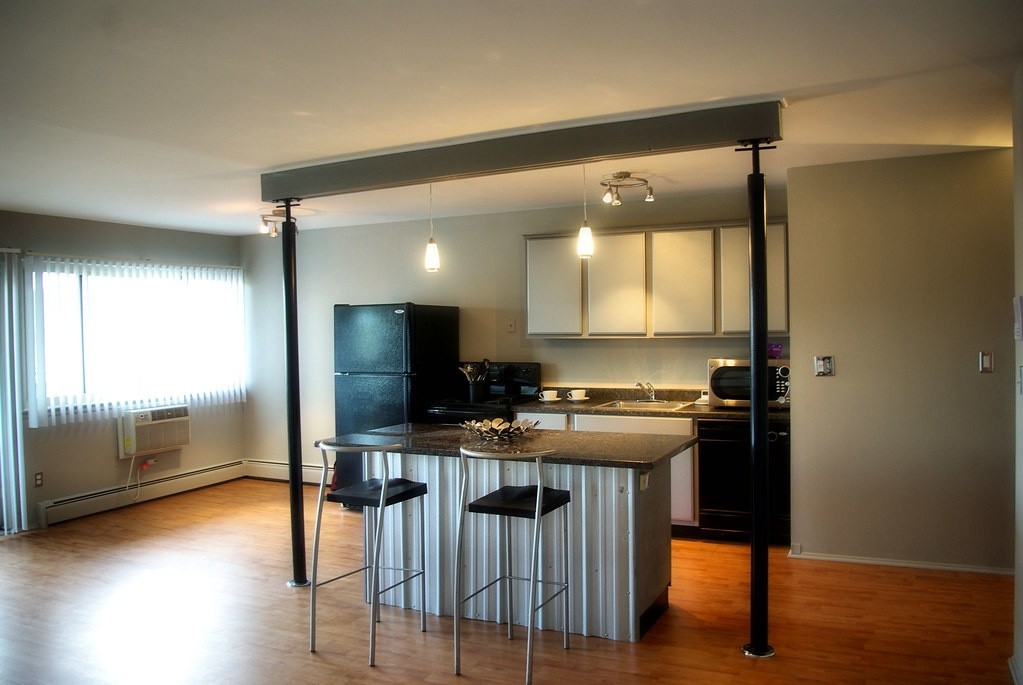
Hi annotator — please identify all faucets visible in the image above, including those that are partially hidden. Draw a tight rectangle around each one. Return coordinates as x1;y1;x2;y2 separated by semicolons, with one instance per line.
634;382;656;400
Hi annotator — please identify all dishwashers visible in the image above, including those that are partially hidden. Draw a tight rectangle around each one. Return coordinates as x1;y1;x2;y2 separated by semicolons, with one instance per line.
697;419;790;540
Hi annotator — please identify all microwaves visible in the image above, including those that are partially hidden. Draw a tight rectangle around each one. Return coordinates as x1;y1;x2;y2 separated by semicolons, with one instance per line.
707;356;790;409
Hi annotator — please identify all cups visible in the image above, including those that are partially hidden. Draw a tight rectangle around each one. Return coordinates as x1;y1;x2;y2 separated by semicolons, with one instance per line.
461;380;482;402
538;390;558;399
567;389;587;399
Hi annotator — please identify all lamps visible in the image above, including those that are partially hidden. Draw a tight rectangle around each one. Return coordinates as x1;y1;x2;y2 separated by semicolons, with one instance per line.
576;161;594;260
600;171;654;205
260;209;297;237
423;182;440;275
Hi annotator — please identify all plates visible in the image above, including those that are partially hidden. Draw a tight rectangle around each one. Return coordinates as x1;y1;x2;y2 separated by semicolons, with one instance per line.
566;397;590;403
538;397;562;404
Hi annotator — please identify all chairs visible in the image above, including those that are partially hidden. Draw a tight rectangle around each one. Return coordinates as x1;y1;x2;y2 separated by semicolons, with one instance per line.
454;447;574;684
310;439;427;667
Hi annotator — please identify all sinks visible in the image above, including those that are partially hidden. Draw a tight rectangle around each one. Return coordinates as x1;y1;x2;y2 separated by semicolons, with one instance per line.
592;400;693;411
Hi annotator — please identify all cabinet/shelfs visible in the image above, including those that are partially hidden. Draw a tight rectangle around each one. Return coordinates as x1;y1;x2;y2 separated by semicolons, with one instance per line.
521;217;791;340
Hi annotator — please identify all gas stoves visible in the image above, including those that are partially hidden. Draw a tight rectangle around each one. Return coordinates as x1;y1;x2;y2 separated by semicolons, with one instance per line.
426;360;541;415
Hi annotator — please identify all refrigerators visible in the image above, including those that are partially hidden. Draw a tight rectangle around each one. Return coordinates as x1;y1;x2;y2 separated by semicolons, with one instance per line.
333;304;460;508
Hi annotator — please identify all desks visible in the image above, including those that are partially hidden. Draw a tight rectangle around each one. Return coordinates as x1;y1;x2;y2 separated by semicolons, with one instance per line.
363;423;699;643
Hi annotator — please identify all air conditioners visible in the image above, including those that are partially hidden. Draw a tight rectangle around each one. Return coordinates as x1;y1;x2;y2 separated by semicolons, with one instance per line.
117;405;192;458
